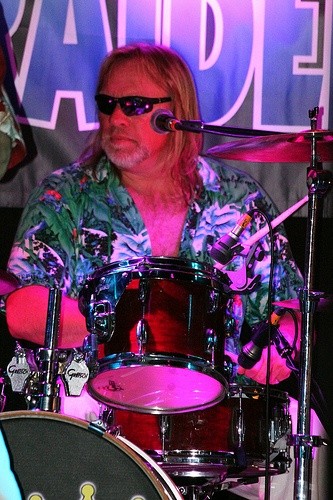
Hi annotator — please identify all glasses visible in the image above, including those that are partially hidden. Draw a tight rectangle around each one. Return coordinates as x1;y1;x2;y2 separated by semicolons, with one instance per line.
95;93;173;117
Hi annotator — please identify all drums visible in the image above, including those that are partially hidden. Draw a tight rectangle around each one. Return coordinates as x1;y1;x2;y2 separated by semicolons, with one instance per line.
222;382;291;479
76;256;232;414
1;410;182;500
101;407;232;479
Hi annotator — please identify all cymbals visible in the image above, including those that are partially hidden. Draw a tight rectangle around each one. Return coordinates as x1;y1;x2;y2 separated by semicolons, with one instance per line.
206;129;333;163
271;296;333;315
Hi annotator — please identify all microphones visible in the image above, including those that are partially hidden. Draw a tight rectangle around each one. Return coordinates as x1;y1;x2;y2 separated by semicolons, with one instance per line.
209;210;255;265
150;108;182;134
238;308;286;370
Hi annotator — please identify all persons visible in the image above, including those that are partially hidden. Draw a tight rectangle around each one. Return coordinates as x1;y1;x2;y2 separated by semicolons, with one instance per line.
0;42;327;500
